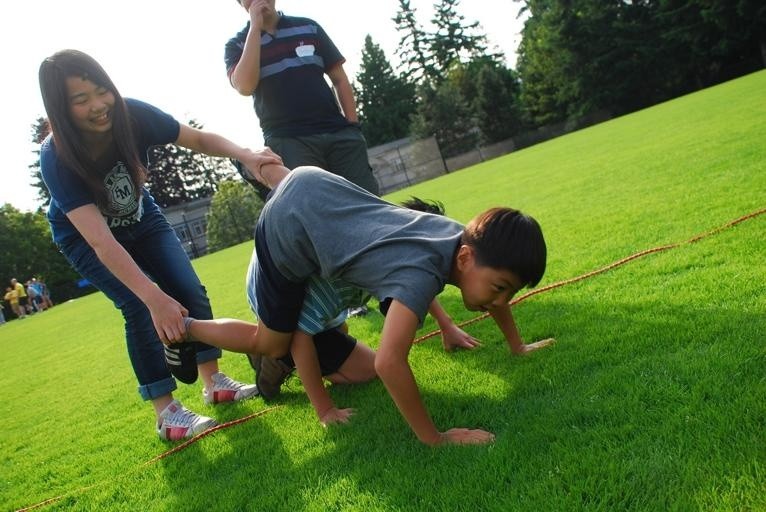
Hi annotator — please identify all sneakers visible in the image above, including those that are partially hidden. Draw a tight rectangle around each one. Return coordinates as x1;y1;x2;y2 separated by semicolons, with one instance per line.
348;305;370;317
248;354;295;404
163;337;198;385
229;156;272;203
155;399;219;442
202;371;259;405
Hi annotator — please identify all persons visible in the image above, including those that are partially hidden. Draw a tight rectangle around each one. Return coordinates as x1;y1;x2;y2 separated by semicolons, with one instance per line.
0;274;53;324
244;195;485;403
38;49;285;441
161;151;558;446
222;0;384;317
